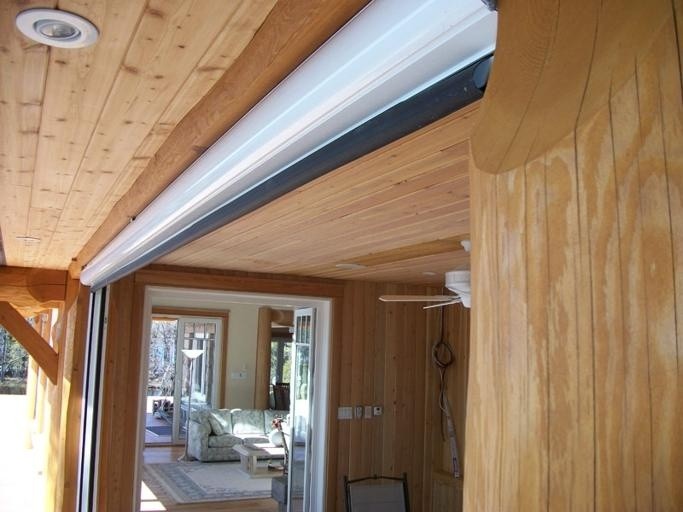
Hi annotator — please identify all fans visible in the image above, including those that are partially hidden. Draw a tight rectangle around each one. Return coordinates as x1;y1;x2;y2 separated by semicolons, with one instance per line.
379;241;471;310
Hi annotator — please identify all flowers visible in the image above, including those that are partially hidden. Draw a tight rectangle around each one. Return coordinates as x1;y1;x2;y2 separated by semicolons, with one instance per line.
271;418;284;430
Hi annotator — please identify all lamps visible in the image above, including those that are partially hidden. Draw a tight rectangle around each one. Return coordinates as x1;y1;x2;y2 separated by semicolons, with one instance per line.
177;349;206;460
18;9;99;48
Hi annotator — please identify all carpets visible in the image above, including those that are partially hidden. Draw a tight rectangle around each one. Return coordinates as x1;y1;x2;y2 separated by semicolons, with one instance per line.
145;426;187;435
142;462;288;504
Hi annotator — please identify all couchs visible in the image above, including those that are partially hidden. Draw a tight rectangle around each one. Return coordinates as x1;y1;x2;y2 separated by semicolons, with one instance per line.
187;408;290;461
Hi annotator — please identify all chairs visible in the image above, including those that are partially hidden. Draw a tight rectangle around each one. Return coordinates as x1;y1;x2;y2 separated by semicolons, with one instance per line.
344;472;411;512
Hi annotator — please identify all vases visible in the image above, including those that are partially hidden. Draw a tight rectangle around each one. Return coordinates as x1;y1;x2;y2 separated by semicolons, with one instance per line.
268;429;284;447
